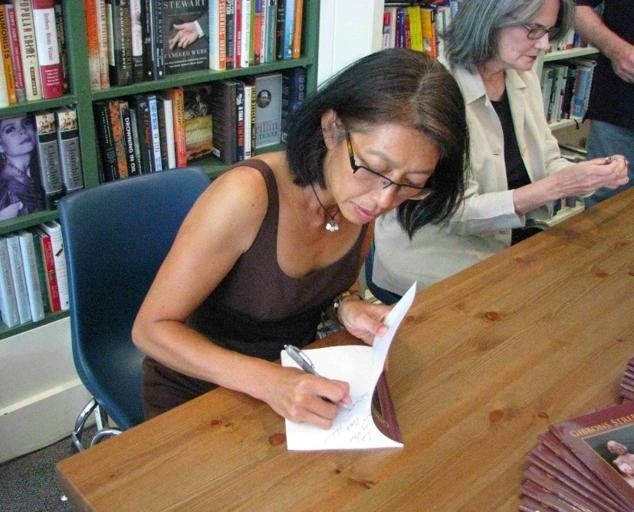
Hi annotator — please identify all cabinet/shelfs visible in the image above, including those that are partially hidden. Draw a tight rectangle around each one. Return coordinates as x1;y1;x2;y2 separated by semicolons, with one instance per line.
0;1;321;464
372;0;605;133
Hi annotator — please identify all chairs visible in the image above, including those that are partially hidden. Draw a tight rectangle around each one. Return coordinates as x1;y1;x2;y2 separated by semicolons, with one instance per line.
56;165;212;459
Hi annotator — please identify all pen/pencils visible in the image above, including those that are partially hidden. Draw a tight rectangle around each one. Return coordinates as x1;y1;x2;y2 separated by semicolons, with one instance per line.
604;156;611;164
283;344;352;413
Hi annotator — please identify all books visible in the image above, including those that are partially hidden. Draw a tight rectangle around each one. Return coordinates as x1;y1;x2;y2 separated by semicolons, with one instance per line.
0;220;71;328
514;353;633;511
0;1;303;106
0;68;309;221
377;1;597;141
272;280;424;451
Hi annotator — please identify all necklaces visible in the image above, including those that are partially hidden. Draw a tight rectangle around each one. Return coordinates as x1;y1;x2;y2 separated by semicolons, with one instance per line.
306;178;343;234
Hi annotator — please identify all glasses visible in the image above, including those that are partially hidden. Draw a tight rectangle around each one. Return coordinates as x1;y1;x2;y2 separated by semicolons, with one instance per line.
508;14;560;41
340;113;435;200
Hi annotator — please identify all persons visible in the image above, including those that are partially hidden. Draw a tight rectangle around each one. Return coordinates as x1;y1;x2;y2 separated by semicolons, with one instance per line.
130;44;469;434
569;0;633;210
361;1;631;306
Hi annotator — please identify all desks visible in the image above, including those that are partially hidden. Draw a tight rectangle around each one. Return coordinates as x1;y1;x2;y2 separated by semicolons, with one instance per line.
52;185;634;510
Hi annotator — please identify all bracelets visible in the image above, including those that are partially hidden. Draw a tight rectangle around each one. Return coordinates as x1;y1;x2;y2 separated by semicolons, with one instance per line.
331;289;361;327
